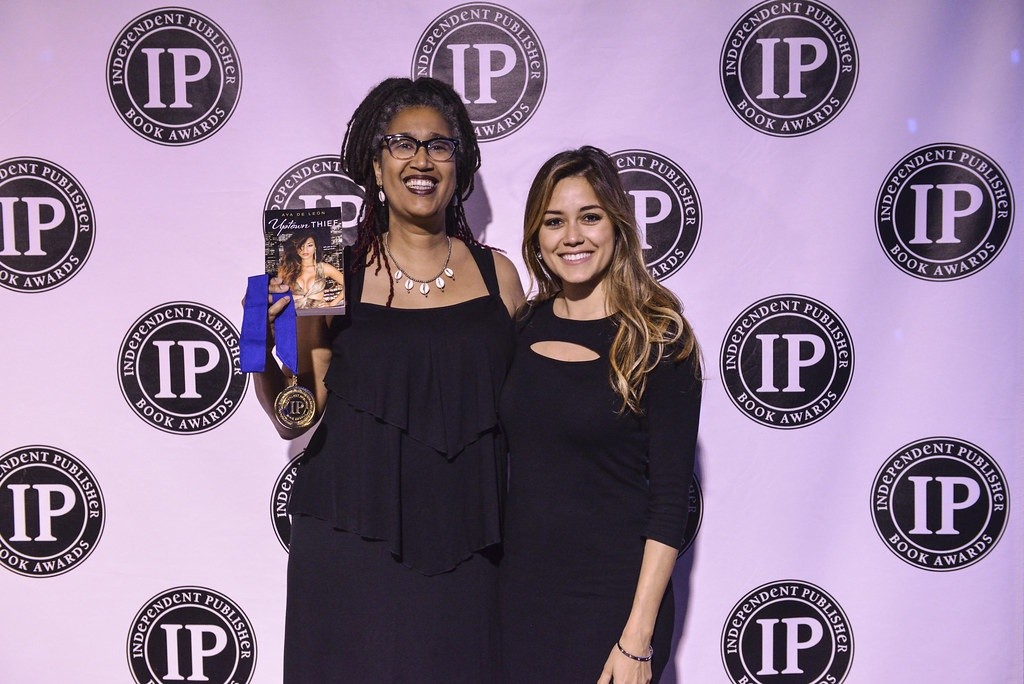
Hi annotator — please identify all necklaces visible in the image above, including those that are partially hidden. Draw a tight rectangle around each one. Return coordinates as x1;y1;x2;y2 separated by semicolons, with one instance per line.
379;234;458;298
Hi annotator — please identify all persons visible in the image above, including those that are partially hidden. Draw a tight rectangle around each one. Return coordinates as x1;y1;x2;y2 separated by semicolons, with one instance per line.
514;145;703;683
251;77;528;683
277;232;345;308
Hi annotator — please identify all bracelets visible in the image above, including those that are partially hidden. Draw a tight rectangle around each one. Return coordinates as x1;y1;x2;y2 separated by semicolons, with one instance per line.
615;639;655;664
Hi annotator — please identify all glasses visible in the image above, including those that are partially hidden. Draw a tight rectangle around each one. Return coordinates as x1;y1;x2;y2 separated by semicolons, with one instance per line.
377;134;461;163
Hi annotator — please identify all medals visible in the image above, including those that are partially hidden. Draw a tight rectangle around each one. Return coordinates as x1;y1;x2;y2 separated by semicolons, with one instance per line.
274;384;316;429
